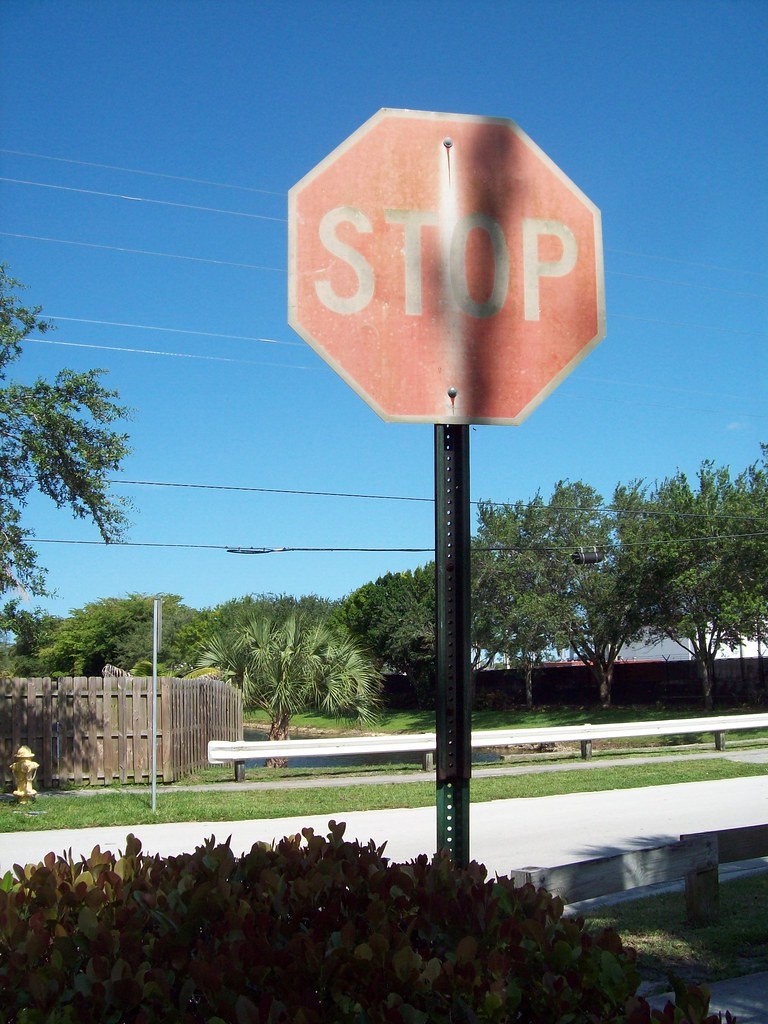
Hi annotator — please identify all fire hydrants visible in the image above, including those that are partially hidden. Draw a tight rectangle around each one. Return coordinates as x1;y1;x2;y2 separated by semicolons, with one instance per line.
9;745;41;807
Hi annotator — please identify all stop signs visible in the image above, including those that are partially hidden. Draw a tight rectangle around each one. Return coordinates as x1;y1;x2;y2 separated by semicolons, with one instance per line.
282;107;607;424
